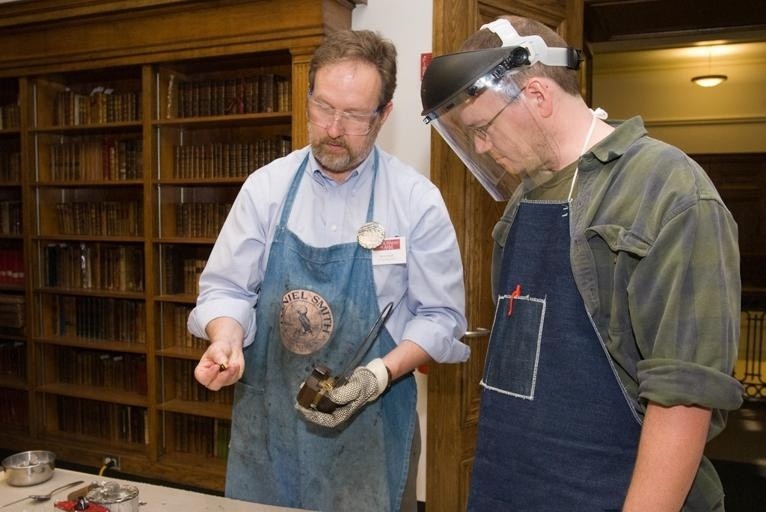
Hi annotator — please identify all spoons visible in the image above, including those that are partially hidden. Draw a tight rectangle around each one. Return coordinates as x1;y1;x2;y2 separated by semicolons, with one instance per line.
30;480;84;501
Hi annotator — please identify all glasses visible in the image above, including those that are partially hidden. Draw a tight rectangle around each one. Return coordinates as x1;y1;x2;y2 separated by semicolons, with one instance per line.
464;87;533;145
306;89;383;135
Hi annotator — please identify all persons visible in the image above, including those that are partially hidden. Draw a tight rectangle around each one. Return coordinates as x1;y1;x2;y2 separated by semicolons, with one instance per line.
417;14;744;511
185;29;473;512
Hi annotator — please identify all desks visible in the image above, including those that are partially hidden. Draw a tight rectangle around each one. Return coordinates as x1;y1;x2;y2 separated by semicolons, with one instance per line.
0;468;317;512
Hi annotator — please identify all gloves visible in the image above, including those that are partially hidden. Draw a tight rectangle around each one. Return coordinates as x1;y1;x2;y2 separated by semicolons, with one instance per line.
294;359;388;428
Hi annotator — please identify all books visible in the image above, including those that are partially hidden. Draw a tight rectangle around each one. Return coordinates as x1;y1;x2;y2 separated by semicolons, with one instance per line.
172;259;235;462
49;91;143;448
172;74;292;118
172;139;292;177
0;104;29;437
176;186;232;238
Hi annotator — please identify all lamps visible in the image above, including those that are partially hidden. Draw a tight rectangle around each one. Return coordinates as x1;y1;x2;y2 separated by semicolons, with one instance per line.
691;75;728;88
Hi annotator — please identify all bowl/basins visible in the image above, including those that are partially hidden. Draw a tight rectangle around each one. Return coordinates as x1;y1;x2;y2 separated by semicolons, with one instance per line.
2;450;56;487
